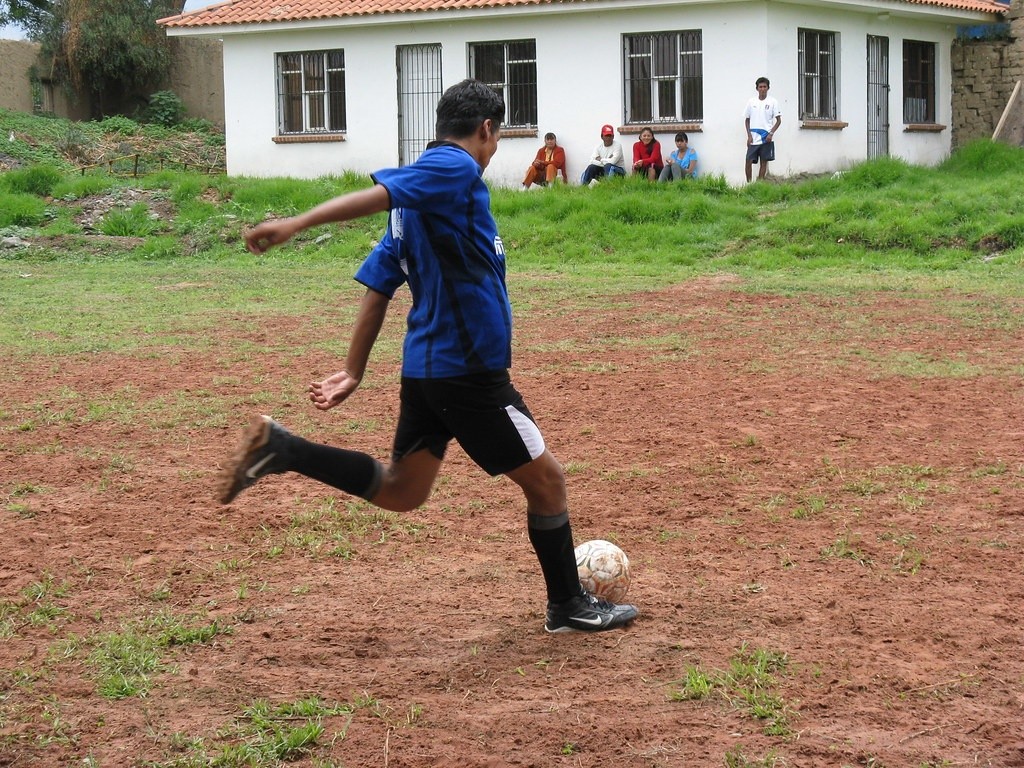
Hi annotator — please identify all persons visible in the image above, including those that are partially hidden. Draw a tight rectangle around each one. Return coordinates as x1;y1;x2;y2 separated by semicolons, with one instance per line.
744;76;781;184
578;124;627;187
219;78;639;633
521;132;568;193
632;127;664;180
658;131;699;182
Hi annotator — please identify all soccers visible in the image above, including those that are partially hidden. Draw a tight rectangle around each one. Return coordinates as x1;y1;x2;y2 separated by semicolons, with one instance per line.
574;539;631;605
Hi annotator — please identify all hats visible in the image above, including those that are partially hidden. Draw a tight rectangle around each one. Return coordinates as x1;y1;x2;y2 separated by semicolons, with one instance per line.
603;125;613;136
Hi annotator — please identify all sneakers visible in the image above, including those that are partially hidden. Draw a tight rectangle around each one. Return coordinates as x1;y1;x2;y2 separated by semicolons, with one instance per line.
214;415;290;505
544;583;639;633
